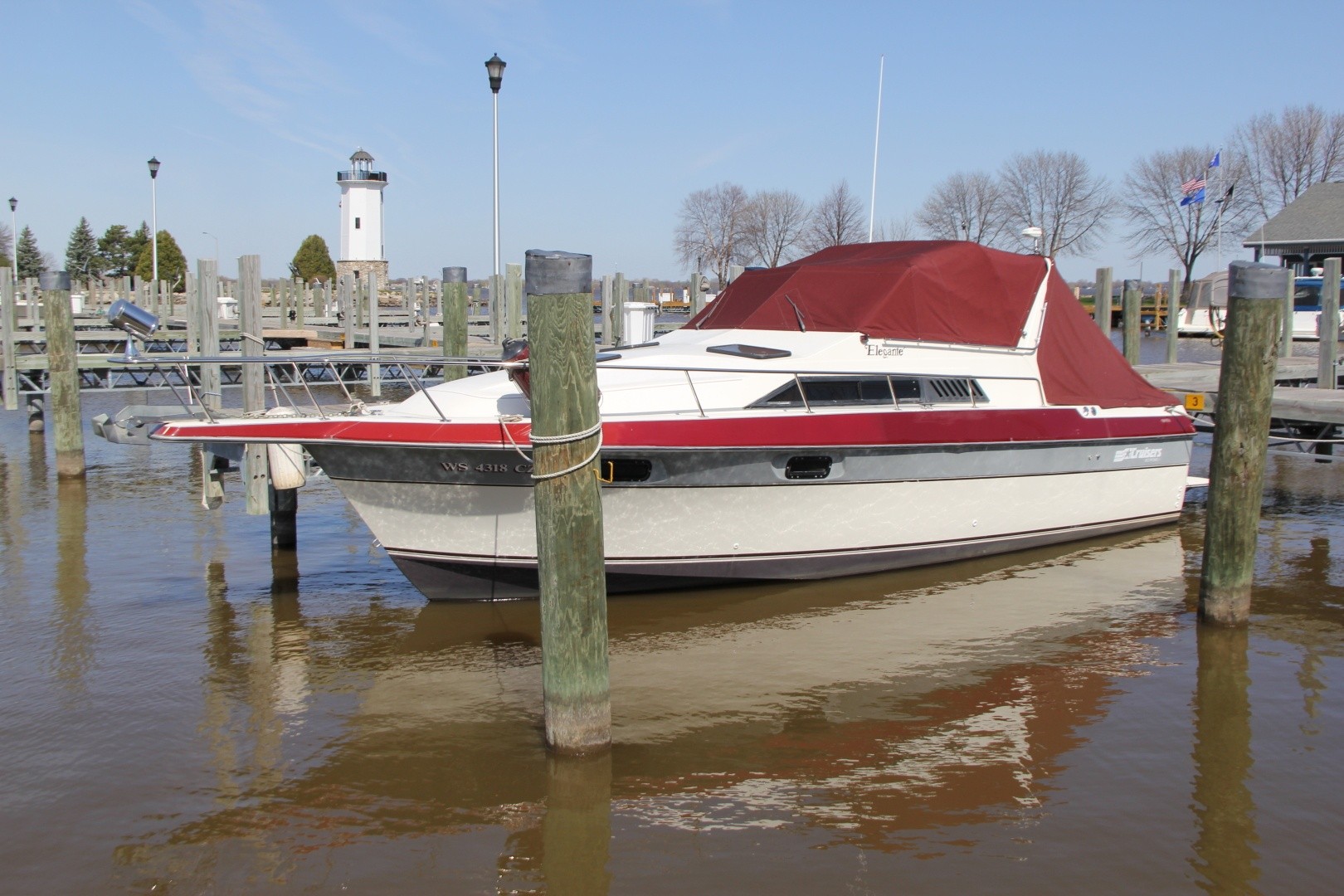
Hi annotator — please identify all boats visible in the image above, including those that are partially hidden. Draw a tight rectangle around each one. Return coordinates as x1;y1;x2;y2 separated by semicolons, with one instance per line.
144;238;1214;604
1279;267;1344;343
1176;269;1229;337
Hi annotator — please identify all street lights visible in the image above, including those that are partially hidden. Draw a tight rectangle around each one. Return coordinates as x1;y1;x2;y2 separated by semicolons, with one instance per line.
147;156;161;329
483;52;506;344
8;196;20;303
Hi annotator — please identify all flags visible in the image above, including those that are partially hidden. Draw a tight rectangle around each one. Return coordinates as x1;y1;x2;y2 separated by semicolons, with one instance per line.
1208;152;1221;168
1180;186;1206;207
1181;170;1206;194
1215;183;1235;203
